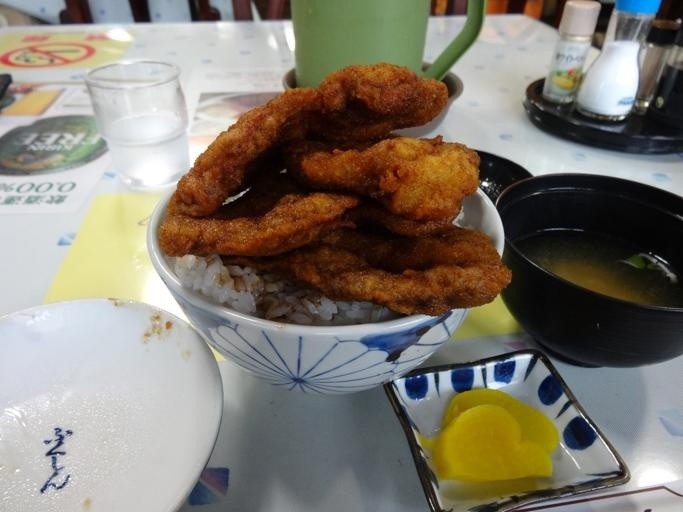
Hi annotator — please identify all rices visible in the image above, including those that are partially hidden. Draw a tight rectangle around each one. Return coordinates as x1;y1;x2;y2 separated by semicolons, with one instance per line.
172;204;466;325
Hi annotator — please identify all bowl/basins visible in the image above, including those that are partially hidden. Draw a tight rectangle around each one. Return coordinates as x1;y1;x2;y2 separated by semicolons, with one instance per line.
493;173;683;368
280;64;463;140
146;188;505;394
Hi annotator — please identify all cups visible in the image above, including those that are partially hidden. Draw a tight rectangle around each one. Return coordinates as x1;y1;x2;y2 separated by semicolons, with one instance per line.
85;60;190;191
289;1;484;89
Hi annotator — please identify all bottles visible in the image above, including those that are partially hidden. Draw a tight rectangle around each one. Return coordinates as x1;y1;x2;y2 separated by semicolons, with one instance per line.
631;20;678;116
654;35;683;112
574;0;661;121
542;0;601;105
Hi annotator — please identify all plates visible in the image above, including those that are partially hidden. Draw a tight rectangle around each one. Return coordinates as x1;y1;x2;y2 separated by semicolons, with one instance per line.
0;299;223;511
384;348;630;511
